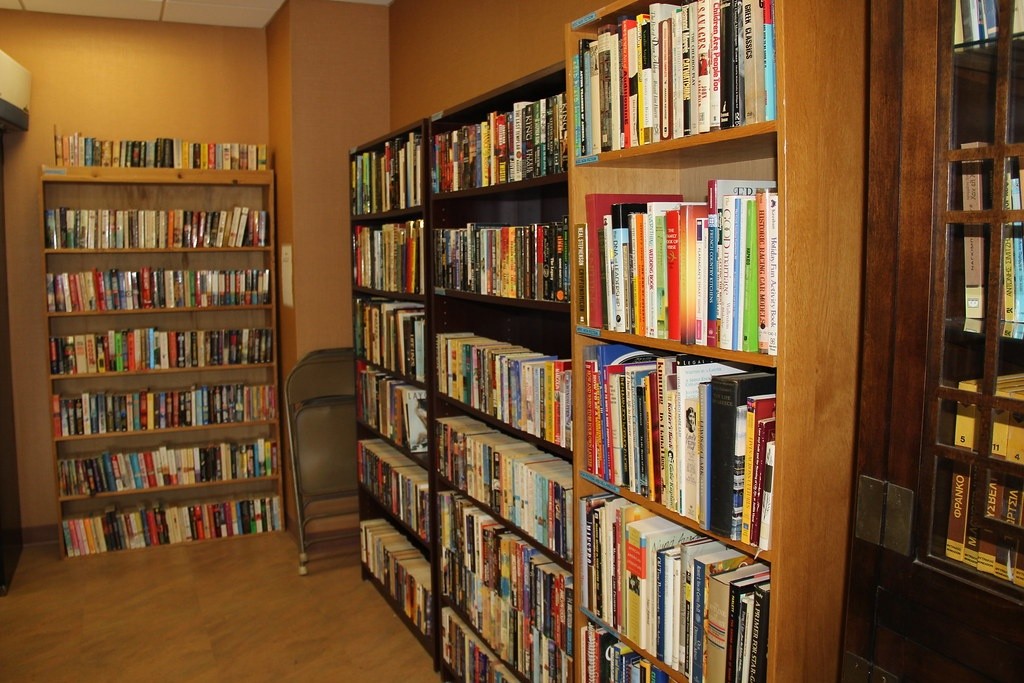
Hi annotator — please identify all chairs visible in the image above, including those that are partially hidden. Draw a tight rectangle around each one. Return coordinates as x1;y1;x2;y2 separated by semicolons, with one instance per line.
285;347;361;575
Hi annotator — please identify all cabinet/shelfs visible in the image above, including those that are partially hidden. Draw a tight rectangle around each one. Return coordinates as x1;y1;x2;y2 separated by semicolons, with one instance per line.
347;115;440;675
38;162;288;559
564;0;840;683
851;0;1023;682
427;60;574;683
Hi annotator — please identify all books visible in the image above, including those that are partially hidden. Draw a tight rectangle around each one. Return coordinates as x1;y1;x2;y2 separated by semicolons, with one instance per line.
571;0;777;158
349;132;432;637
46;269;270;312
49;327;272;374
574;180;777;356
52;383;275;437
435;416;574;683
55;132;267;170
945;374;1024;587
579;492;770;683
46;207;266;248
580;622;677;683
57;439;278;497
961;0;998;50
435;332;573;452
63;491;282;557
961;141;1024;320
583;345;776;551
433;215;570;303
431;91;568;193
